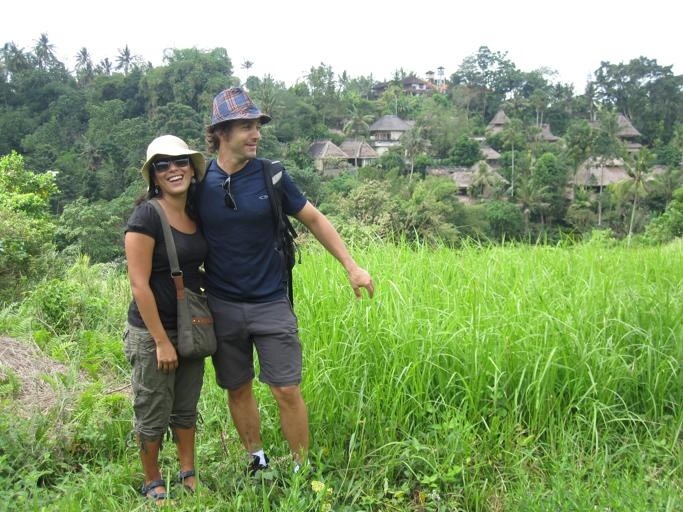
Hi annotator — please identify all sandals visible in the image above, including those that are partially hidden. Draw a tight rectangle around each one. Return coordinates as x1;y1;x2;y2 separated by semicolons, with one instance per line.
141;470;214;508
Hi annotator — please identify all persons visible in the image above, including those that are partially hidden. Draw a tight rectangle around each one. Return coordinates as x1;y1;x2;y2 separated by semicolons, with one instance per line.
191;86;377;490
121;131;218;503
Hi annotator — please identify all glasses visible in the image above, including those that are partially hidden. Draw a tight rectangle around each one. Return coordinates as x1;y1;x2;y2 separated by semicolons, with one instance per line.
222;176;238;211
151;156;191;173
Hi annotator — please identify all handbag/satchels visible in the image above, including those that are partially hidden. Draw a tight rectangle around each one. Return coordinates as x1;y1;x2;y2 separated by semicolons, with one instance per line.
176;288;217;358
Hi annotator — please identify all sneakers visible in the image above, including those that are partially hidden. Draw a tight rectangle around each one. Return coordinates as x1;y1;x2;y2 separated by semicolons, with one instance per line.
232;453;273;494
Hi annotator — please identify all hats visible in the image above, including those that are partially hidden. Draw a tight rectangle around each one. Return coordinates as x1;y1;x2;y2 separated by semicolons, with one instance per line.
141;134;206;183
210;86;272;128
